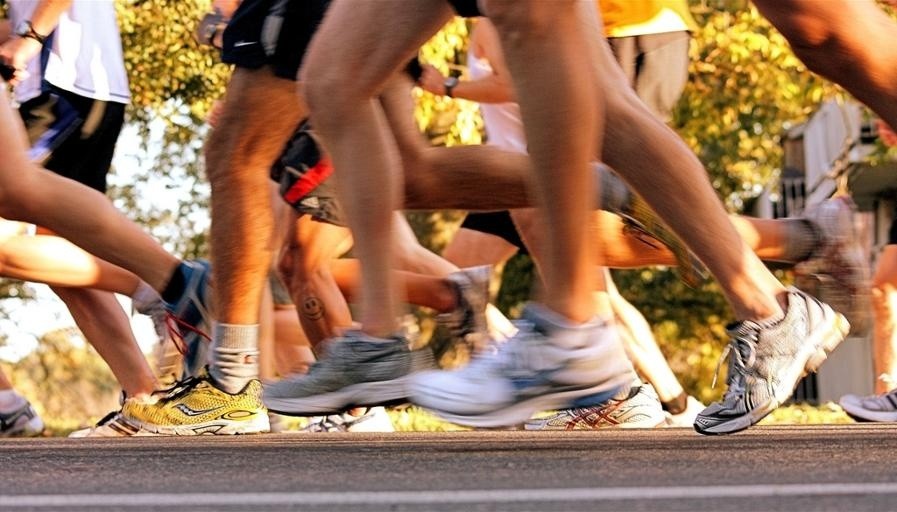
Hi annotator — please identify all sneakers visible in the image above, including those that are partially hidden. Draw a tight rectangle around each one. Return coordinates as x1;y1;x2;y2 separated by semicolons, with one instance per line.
130;294;183;387
122;364;270;434
164;259;215;376
3;401;44;433
693;284;851;432
261;208;683;434
70;387;160;439
786;199;871;341
619;173;708;285
840;392;896;425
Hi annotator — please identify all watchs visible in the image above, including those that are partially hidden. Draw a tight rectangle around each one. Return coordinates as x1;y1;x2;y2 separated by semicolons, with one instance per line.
16;19;47;45
444;77;458;98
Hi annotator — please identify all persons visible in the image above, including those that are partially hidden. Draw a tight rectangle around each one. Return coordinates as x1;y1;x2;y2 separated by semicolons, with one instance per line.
2;0;212;441
758;1;897;434
128;1;851;435
